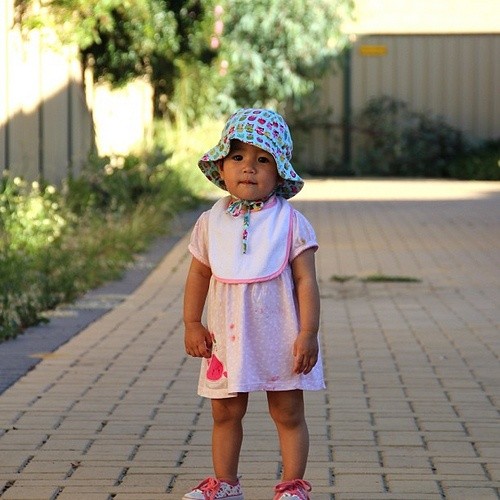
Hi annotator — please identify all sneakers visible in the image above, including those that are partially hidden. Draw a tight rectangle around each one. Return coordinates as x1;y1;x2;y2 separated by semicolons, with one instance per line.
273;479;312;500
182;477;243;500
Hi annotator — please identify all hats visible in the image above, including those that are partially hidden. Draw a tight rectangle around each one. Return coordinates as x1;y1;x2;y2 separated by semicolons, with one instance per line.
198;108;304;200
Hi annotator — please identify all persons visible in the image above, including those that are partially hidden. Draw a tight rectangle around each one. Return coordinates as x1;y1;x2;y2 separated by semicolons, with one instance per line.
182;108;326;500
